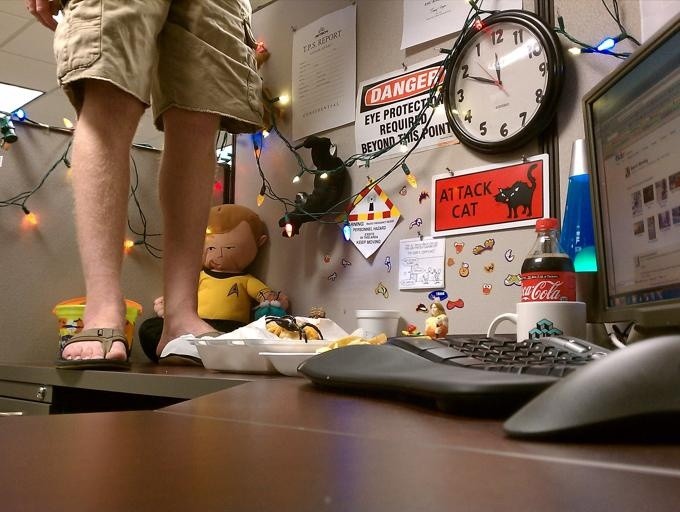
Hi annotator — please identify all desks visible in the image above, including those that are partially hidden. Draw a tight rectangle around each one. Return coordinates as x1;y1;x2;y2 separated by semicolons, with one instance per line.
0;359;680;512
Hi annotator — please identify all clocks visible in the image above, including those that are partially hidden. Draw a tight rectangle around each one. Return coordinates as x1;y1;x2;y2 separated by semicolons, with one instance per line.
443;8;565;155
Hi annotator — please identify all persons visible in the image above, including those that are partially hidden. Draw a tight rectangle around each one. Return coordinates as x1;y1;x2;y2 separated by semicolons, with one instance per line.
424;299;451;339
137;205;289;360
22;0;271;372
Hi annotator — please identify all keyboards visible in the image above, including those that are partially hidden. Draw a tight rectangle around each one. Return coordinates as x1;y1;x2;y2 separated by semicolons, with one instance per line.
295;335;614;414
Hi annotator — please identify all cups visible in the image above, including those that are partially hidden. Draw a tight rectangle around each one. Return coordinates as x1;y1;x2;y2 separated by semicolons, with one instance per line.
484;299;589;343
355;308;402;340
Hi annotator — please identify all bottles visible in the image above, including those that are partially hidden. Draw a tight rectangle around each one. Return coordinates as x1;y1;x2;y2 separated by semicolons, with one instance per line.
518;216;580;302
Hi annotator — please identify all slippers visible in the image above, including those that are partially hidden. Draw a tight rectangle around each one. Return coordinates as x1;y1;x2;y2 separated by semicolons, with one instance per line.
54;328;132;370
158;332;227;367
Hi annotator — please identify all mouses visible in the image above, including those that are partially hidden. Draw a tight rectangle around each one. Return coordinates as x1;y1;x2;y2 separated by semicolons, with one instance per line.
503;333;680;439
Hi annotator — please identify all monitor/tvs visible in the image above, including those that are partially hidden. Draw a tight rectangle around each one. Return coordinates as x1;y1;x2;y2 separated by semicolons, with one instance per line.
580;12;680;331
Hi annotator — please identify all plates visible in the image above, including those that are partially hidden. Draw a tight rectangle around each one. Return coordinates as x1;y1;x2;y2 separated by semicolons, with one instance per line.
255;349;320;376
